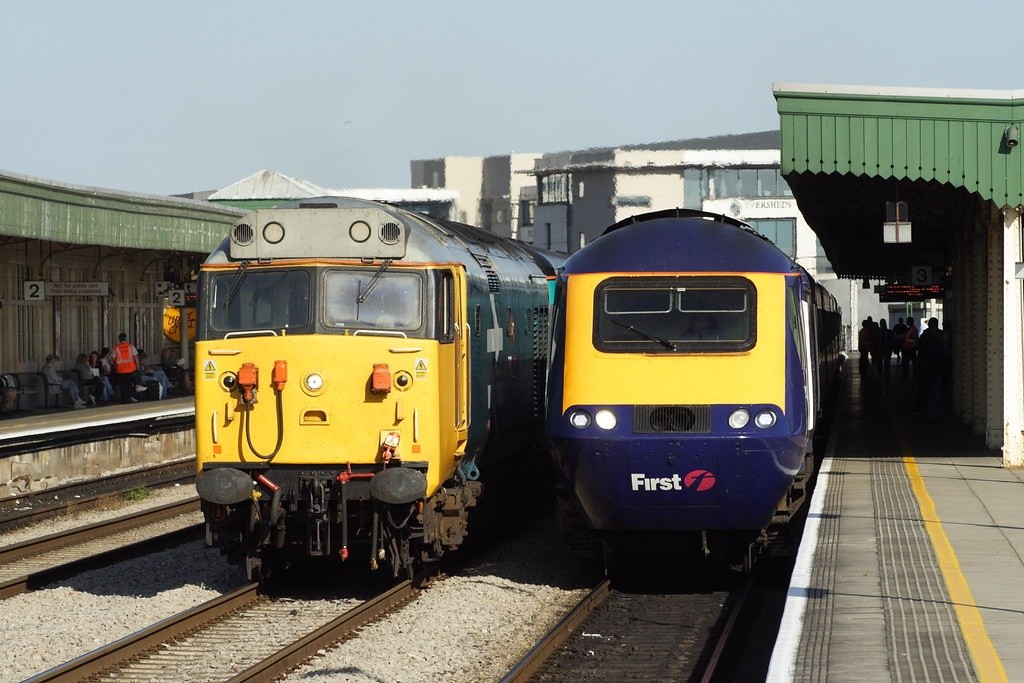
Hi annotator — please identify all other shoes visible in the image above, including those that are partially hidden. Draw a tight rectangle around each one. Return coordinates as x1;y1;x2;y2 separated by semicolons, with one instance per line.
77;397;87;405
128;396;138;403
74;402;87;410
136;384;148;393
88;394;97;408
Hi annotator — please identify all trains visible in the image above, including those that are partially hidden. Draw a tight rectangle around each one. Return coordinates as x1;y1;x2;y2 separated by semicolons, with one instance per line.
543;207;843;584
194;197;576;587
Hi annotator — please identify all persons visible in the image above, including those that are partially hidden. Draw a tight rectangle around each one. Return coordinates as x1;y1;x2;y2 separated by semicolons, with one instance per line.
858;314;952;415
75;334;189;407
40;354;86;409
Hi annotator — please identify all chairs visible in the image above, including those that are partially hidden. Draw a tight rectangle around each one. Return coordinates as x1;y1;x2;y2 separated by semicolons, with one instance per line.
37;371;71;410
69;368;98;404
0;373;38;413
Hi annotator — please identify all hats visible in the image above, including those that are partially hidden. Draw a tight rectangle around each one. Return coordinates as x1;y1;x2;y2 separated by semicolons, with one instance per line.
45;353;54;364
